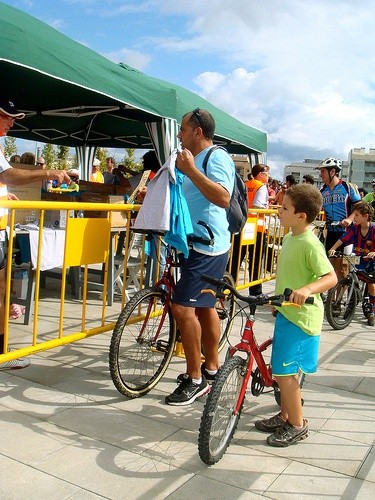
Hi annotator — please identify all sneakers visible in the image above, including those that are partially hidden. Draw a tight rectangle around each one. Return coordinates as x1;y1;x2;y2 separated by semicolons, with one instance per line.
176;362;221;386
254;412;289;433
165;373;211;407
266;419;310;448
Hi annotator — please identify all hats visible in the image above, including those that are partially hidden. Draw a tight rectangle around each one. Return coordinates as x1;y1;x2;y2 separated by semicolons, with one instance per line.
0;98;26;120
92;158;101;167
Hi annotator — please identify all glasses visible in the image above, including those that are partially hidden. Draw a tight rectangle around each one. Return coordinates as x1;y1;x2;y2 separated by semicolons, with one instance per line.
142;161;145;164
0;114;16;122
261;172;270;176
302;180;306;183
106;161;110;164
192;106;205;130
36;162;45;167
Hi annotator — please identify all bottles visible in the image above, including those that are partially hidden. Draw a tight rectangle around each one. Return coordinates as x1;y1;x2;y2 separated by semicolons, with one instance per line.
77;210;84;218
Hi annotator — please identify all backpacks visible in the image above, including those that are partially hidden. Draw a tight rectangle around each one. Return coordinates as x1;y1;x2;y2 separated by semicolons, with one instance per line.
201;145;249;235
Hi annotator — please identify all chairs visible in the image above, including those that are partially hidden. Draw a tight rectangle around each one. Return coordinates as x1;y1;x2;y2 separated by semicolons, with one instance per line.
98;232;151;301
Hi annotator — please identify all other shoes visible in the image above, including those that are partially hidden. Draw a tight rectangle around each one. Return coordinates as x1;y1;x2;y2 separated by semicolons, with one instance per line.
348;295;357;308
366;303;375;326
0;354;31;371
331;302;341;317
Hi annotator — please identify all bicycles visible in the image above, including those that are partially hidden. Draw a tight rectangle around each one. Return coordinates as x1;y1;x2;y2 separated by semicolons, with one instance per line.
108;218;238;400
325;250;375;331
196;271;314;465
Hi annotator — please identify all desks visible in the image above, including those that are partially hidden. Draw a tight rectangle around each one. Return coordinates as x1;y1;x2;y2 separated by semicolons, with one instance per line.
9;226;131;326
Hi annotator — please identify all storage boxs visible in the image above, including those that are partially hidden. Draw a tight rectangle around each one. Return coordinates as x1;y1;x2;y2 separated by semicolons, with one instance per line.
45;209;74;228
42;192;81;202
7;159;43;227
81;193;128;227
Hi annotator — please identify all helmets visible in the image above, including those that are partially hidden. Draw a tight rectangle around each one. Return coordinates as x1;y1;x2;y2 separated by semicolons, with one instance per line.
314;157;342;170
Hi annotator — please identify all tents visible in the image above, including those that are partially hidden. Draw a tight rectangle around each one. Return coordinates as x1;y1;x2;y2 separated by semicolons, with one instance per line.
0;1;268;287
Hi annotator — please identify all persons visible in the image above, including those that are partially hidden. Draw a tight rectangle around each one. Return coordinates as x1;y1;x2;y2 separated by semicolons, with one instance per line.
164;107;236;406
255;182;339;448
0;99;375;328
